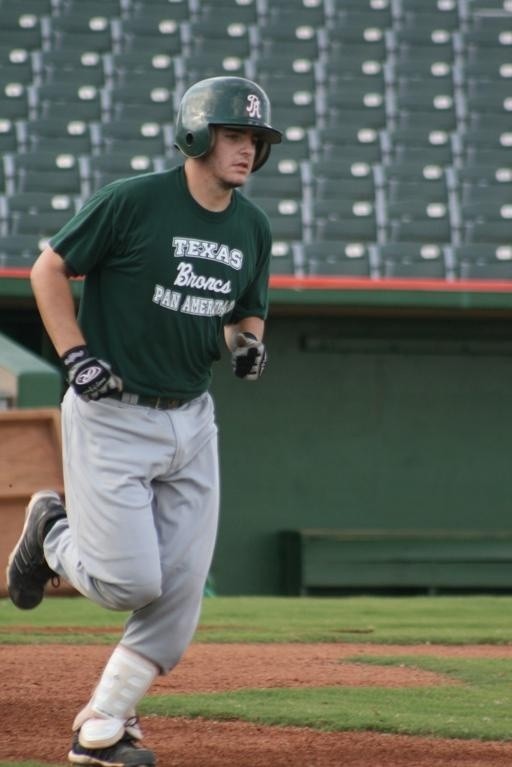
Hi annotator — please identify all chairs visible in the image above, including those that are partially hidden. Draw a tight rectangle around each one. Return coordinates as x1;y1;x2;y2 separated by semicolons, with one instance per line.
0;0;511;279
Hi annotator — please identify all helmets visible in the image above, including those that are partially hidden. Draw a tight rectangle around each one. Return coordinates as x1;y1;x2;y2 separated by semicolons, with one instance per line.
173;75;283;173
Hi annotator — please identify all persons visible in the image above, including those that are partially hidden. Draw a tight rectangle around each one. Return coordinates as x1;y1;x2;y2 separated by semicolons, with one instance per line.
0;73;287;767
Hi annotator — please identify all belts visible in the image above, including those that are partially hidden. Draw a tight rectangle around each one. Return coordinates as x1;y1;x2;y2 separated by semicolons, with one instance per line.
105;390;189;413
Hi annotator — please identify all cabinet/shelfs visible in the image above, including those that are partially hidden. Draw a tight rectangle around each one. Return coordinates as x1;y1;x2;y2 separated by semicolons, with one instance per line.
0;406;65;597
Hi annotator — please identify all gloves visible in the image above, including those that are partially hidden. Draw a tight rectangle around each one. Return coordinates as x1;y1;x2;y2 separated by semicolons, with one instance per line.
230;330;269;382
61;344;124;402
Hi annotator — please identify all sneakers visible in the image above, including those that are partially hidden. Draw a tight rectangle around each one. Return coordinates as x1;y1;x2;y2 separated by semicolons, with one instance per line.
67;707;157;767
6;489;68;610
